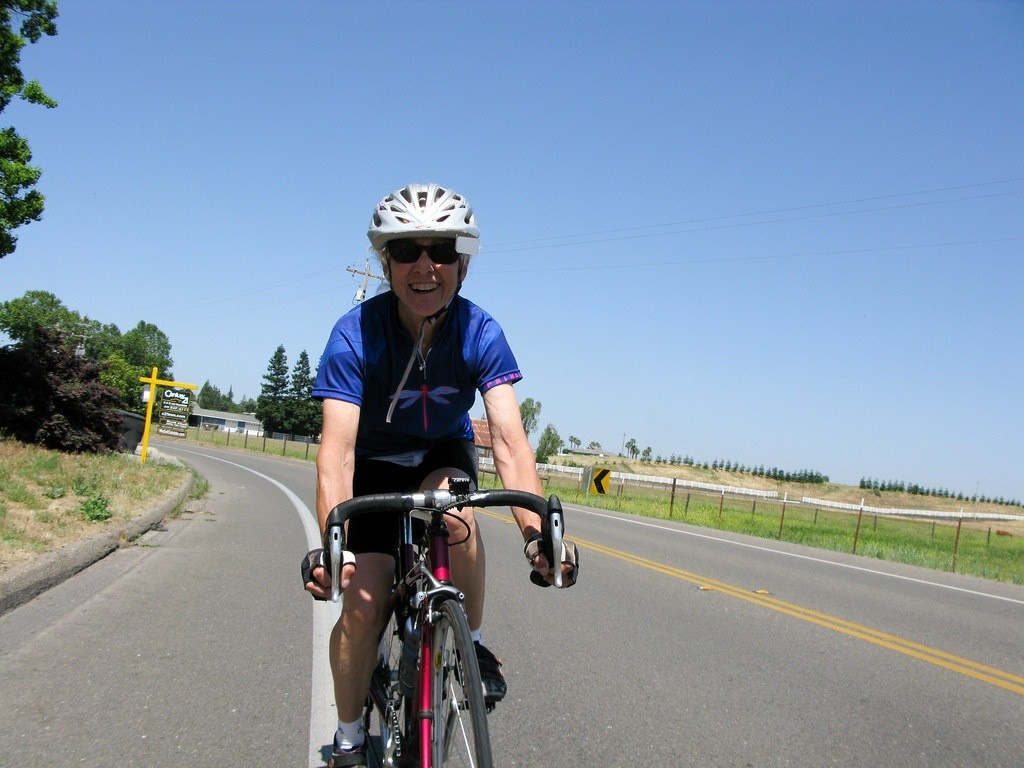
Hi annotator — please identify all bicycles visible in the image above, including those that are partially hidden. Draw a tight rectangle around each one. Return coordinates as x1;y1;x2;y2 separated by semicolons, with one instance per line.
320;486;577;768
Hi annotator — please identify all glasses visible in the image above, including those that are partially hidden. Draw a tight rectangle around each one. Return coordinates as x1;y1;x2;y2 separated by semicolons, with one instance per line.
382;239;463;264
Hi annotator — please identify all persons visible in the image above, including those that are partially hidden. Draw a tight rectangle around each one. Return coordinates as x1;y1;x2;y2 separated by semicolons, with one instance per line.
301;184;580;768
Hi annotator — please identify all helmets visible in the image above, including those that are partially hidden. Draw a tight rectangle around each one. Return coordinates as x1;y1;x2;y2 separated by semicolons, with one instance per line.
368;184;480;265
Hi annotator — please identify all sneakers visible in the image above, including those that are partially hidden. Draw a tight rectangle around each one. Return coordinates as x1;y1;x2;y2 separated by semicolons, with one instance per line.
328;732;372;768
452;643;508;702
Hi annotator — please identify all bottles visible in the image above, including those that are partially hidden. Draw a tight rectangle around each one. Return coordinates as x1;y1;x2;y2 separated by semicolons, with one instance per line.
399;601;425;696
